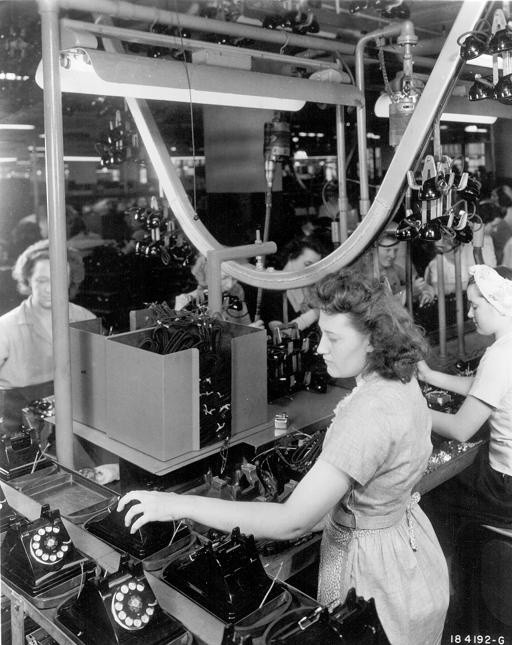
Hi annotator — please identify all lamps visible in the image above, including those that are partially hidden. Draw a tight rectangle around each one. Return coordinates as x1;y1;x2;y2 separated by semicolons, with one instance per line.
375;71;512;125
34;16;366;113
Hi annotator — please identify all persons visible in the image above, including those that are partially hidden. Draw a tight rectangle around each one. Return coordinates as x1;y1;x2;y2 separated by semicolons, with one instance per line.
1;238;100;391
377;181;511;307
416;263;511;521
115;271;452;644
12;201;150;300
315;185;353;221
245;240;325;344
173;254;267;332
